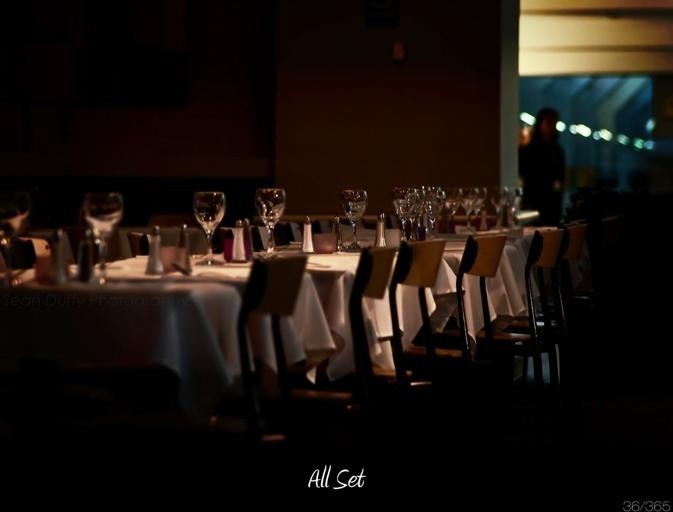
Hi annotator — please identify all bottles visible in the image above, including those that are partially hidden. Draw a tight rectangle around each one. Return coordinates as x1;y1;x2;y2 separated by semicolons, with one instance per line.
143;223;167;275
232;219;247;263
78;229;106;284
176;223;192;274
302;216;315;254
49;226;69;285
478;204;489;231
374;213;388;247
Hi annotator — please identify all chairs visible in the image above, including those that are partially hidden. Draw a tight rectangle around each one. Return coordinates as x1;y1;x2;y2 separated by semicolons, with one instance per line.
192;253;306;469
471;228;571;398
407;229;510;418
510;225;594;372
286;244;394;452
339;241;449;440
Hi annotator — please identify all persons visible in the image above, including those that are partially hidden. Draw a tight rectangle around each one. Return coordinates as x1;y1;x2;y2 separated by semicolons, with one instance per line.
518;107;567;228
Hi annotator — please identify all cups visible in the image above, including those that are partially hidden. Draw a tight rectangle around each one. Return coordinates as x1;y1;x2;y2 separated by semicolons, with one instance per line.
465;186;487;229
84;190;124;286
339;188;368;248
254;188;286;256
390;184;447;240
492;185;523;229
446;185;462;231
192;191;226;266
